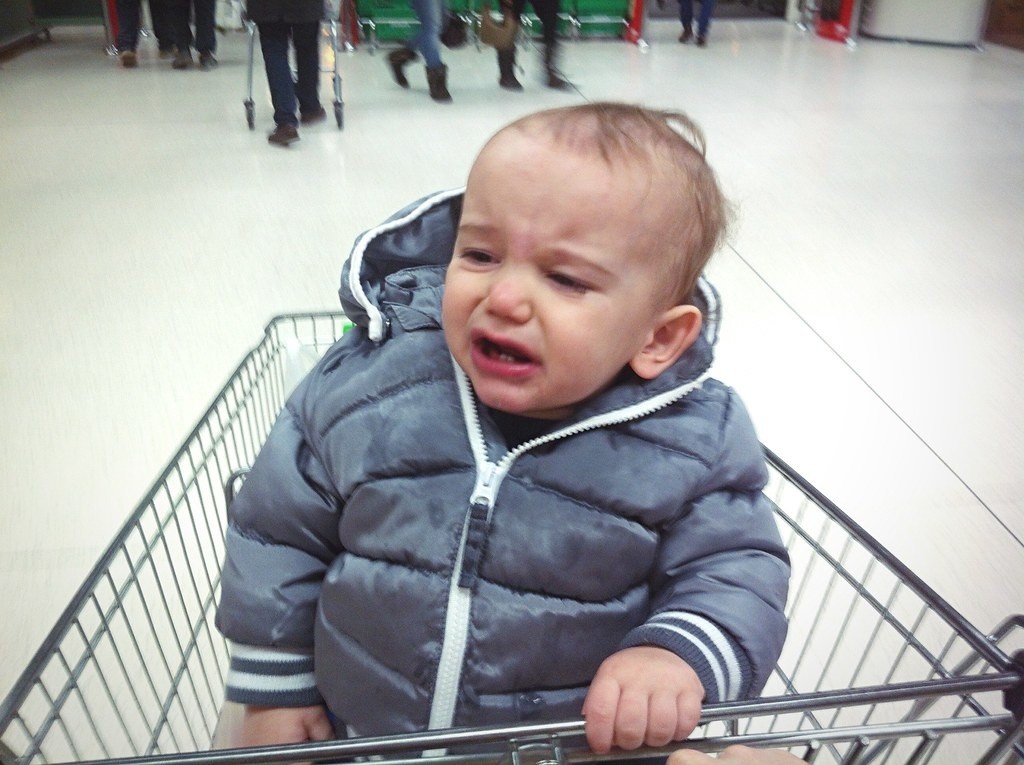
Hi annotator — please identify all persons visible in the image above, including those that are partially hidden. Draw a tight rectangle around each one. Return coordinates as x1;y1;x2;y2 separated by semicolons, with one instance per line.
215;102;811;765
389;0;452;100
115;0;218;71
246;0;326;145
678;0;717;44
498;0;567;89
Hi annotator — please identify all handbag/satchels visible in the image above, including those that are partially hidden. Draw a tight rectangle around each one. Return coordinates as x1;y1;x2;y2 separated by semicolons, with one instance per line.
476;0;516;48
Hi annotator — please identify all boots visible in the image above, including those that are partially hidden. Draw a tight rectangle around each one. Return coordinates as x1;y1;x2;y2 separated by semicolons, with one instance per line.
499;50;524;91
426;64;451;102
389;48;417;88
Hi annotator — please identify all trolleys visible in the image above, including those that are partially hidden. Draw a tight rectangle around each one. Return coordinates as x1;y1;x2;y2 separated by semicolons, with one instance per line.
243;0;345;131
0;310;1023;765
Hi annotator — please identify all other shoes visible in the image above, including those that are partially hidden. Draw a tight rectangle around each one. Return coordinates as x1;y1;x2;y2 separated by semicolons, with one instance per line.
698;35;705;44
679;23;692;42
301;106;326;125
120;46;138;68
172;44;192;68
200;50;218;69
161;47;174;60
269;122;299;143
